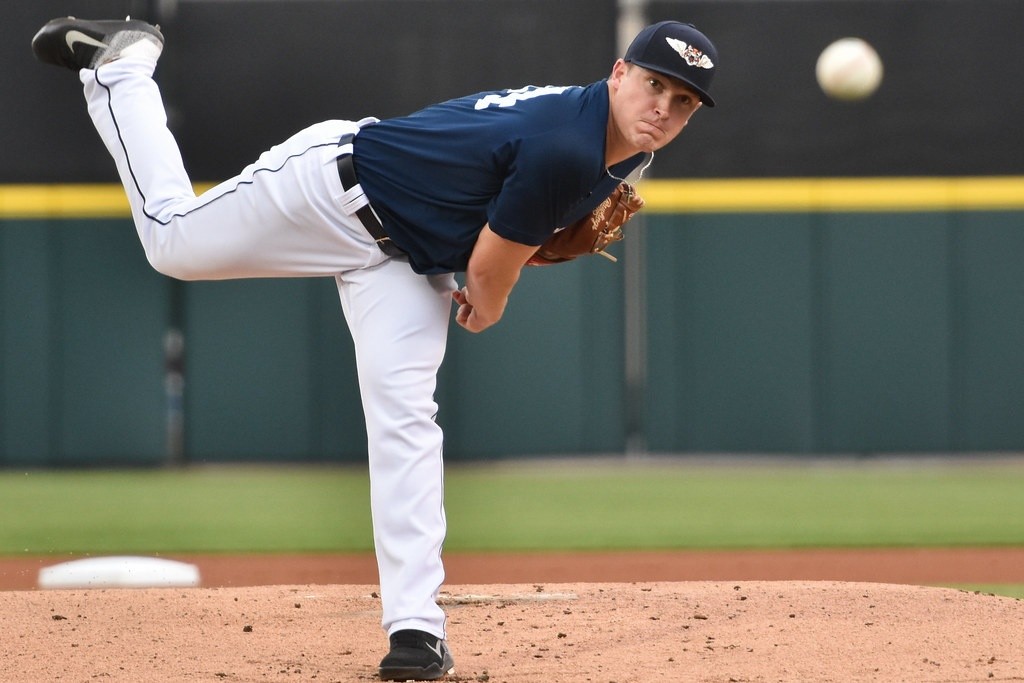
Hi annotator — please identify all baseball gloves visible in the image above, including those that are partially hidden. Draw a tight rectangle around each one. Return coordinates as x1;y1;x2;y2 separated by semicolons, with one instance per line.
524;181;646;267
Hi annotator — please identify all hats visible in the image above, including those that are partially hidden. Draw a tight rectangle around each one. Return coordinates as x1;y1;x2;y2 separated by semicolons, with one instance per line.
625;21;717;107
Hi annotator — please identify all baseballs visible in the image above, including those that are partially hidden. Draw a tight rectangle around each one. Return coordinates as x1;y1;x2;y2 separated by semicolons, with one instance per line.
814;36;884;104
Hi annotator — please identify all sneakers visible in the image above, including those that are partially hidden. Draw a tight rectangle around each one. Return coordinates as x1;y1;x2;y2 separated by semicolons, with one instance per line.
379;629;454;681
31;15;164;71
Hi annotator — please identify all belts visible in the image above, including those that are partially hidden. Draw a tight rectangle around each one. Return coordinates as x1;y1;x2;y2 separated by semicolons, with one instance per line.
337;133;407;257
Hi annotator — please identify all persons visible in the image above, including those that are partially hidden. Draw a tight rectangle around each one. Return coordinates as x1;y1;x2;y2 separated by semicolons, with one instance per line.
31;15;718;681
